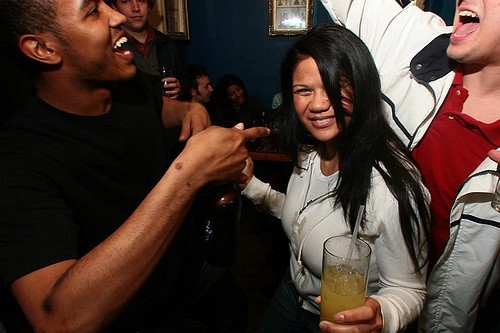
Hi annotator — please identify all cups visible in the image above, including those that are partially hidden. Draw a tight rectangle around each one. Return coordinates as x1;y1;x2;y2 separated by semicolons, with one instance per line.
320;235;372;323
159;65;174;97
492;177;499;212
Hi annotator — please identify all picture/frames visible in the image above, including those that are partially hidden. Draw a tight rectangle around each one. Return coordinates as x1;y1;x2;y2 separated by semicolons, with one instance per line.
269;0;314;36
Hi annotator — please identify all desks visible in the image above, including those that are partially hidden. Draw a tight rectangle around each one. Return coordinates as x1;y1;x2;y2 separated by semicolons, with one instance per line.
226;151;300;235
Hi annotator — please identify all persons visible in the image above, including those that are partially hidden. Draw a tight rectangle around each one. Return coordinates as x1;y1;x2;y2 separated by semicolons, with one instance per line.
320;0;500;333
0;0;271;333
238;23;433;333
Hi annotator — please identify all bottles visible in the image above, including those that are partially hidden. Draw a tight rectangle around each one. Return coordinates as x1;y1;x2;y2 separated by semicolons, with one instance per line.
260;111;272;128
